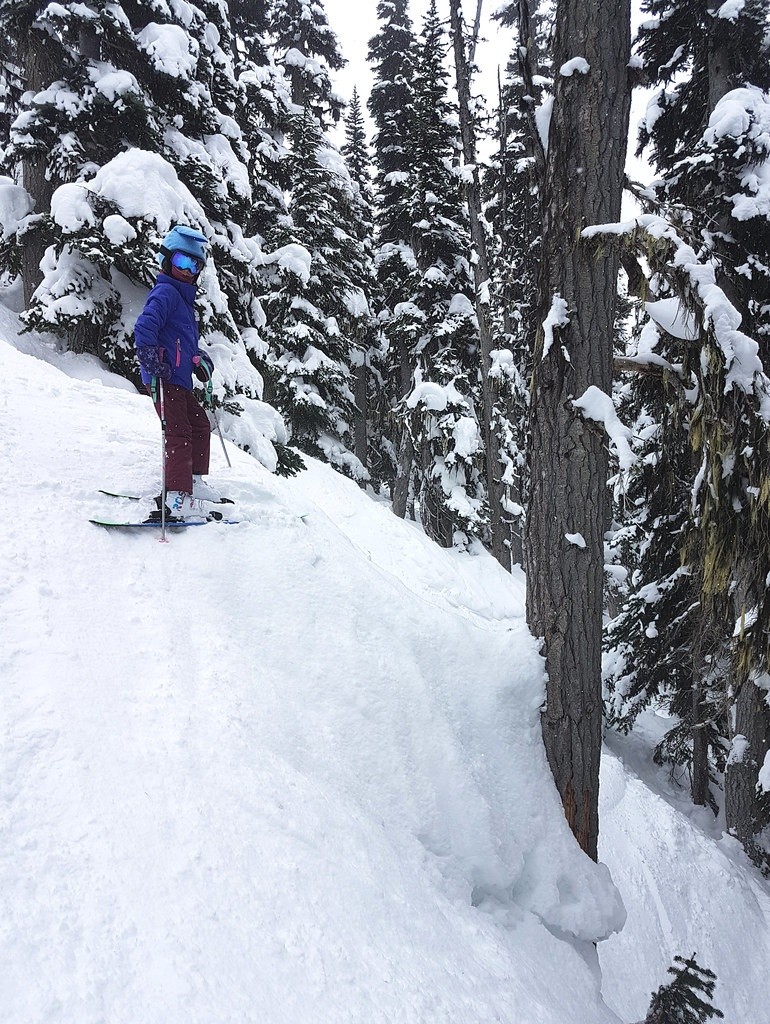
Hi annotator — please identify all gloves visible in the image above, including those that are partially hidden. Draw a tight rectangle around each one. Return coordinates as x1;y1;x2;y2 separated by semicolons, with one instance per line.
138;346;174;382
194;348;214;382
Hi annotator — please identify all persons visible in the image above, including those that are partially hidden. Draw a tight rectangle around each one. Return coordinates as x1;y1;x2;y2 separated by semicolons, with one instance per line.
135;225;234;520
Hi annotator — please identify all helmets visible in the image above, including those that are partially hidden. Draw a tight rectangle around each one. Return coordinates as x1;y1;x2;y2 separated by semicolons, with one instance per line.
159;226;210;263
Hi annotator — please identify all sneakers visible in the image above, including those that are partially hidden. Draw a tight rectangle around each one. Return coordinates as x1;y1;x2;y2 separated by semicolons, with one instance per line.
164;491;223;521
192;474;222;503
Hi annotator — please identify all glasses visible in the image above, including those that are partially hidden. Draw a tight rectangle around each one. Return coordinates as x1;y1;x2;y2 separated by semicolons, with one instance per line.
170;249;204;275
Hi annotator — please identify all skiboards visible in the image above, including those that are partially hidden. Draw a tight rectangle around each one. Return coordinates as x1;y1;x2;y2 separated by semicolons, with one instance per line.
85;488;311;528
